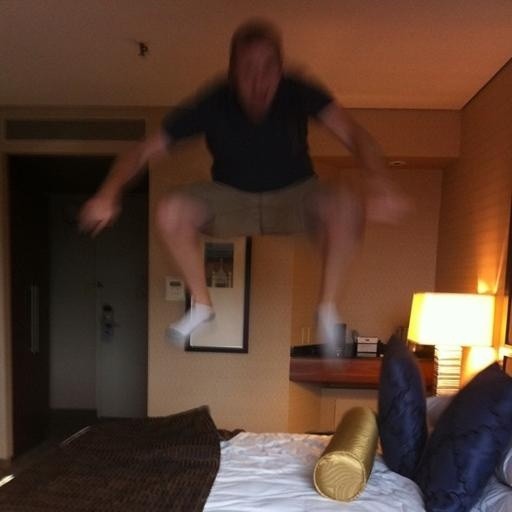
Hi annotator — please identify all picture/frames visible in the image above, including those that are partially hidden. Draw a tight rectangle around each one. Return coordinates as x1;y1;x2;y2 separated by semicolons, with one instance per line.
184;229;251;354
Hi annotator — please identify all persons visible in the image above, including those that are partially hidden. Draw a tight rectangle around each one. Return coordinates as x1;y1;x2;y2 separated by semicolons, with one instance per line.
80;19;413;373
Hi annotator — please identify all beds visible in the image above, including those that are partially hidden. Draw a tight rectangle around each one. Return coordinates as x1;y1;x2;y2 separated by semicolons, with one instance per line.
0;346;512;512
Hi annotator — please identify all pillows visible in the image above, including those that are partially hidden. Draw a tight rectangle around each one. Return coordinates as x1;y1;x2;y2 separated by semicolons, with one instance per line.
413;361;512;511
377;334;428;476
312;406;379;503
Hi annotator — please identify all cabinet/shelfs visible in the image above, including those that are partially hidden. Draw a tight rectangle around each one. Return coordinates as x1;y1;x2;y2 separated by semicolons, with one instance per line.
6;155;49;456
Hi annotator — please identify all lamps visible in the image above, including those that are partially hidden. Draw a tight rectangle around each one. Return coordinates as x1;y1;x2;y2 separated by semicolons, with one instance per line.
402;292;496;398
